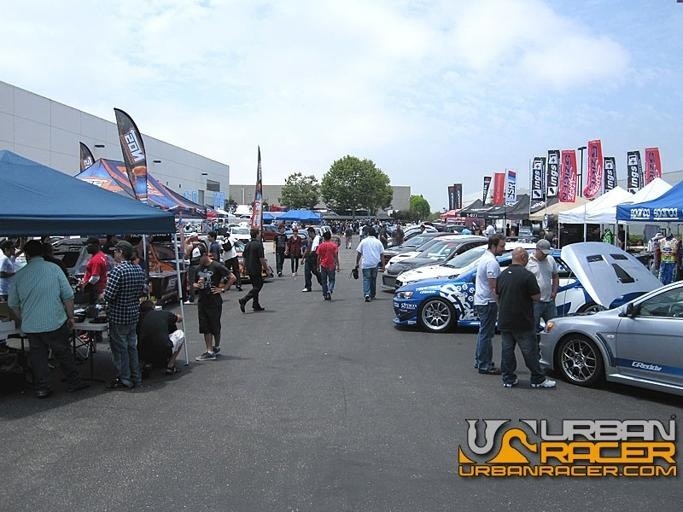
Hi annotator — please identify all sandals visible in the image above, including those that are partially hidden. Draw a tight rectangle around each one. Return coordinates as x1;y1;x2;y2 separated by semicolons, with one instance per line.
143;362;152;371
164;365;177;375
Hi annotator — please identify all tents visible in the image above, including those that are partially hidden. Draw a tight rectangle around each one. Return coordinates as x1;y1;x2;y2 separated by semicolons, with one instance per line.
557;177;673;249
0;148;189;367
529;197;593;221
72;158;228;252
275;210;322;223
262;213;275;221
440;193;540;235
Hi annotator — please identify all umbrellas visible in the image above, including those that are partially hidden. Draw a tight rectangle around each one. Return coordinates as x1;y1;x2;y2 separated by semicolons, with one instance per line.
613;179;683;247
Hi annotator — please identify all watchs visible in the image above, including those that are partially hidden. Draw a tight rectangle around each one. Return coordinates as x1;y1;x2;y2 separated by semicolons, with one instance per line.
221;288;224;291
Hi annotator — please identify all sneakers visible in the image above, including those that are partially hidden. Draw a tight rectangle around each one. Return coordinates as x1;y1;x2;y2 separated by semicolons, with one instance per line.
214;345;222;353
504;380;520;387
292;271;298;277
184;300;195;306
532;379;557;388
66;382;90;390
363;294;372;302
46;388;53;397
373;296;376;299
195;352;217;362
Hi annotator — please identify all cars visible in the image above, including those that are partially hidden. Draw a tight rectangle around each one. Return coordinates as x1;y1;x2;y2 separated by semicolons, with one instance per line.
48;238;115;307
260;225;279;242
394;242;555;291
402;225;437;240
232;228;252;244
384;235;485;270
392;250;599;334
539;242;683;398
283;226;333;258
381;237;492;292
381;231;454;264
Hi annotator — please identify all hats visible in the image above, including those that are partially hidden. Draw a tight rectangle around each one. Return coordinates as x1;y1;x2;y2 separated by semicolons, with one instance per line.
82;237;100;245
109;241;133;255
537;239;552;255
190;243;206;260
190;233;199;238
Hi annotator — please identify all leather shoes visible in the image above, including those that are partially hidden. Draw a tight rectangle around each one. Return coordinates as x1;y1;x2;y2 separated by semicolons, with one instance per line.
238;297;248;313
475;361;496;369
479;365;501;376
301;287;311;292
105;381;129;390
252;304;266;312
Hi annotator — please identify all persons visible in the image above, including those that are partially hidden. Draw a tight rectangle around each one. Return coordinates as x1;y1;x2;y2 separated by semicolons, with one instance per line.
213;220;219;235
358;224;365;241
239;229;271;312
99;240;145;388
618;224;625;250
301;227;323;292
406;221;412;227
495;247;556;388
473;235;506;375
449;229;454;233
41;236;52;244
44;244;69;281
331;227;341;272
506;223;511;236
485;221;496;238
207;232;221;262
420;225;427;234
0;239;24;334
218;233;242;291
301;223;317;228
372;222;380;239
657;228;678;285
323;219;406;233
454;229;458;233
601;228;610;242
7;240;91;397
651;232;664;279
472;220;482;235
271;221;298;229
136;301;185;373
515;223;519;236
524;239;560;332
184;233;207;304
285;229;303;277
272;226;288;276
391;225;405;247
191;247;237;361
80;237;107;342
183;226;192;232
413;221;416;226
380;224;390;250
344;225;353;249
316;231;340;299
197;224;202;233
462;226;471;235
355;226;385;302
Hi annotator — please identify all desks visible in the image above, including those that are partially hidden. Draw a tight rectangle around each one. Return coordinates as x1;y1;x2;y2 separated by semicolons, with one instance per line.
7;306;163;383
